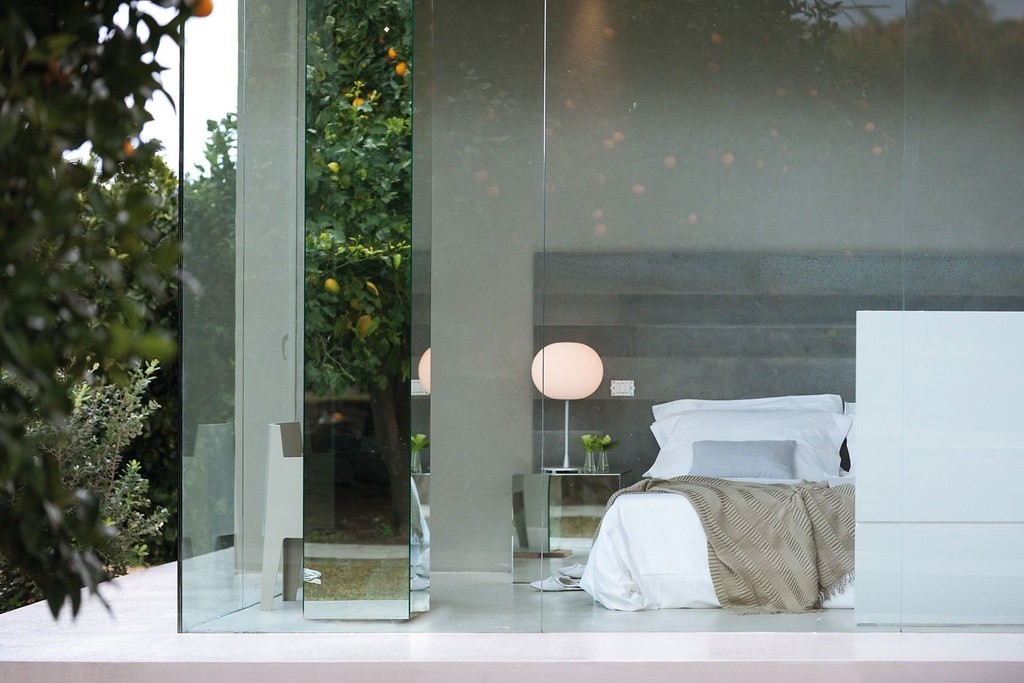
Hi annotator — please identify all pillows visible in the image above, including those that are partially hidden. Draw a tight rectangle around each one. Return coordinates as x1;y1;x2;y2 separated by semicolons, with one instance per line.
652;393;844;415
688;439;797;479
844;402;855;480
642;408;853;484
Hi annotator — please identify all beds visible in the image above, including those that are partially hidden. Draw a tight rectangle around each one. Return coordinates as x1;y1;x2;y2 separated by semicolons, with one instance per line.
579;475;857;617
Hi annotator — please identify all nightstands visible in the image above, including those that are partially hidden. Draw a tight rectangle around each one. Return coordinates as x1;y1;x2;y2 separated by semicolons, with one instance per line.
521;473;621;558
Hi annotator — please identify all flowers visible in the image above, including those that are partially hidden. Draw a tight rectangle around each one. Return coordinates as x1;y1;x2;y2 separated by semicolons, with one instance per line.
595;434;621;451
574;432;599;451
410;434;429;451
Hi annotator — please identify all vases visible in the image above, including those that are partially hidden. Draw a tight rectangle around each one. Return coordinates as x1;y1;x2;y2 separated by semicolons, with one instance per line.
583;451;596;474
596;450;609;474
410;452;422;475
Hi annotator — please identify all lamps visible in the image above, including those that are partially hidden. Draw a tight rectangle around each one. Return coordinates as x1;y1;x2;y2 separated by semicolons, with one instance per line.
531;341;604;473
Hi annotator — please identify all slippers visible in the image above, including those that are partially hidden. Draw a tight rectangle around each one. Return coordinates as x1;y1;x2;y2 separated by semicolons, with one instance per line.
529;574;585;592
557;562;586;578
